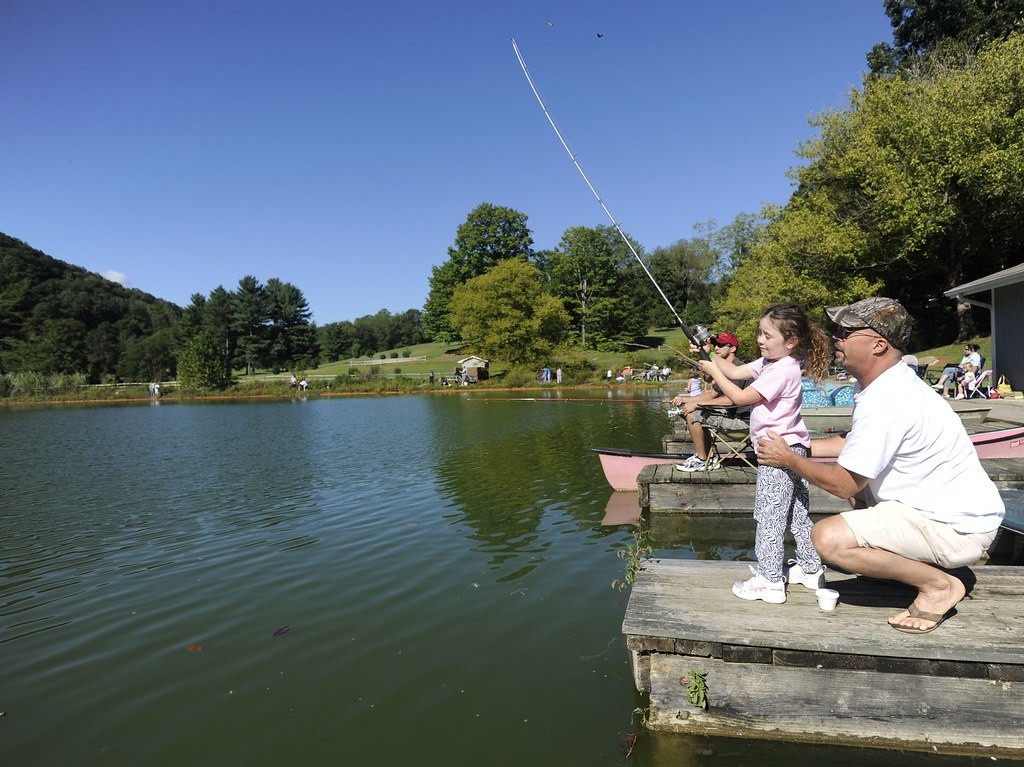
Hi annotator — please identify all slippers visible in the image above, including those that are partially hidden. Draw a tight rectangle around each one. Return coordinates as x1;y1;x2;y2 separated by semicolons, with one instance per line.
891;602;947;634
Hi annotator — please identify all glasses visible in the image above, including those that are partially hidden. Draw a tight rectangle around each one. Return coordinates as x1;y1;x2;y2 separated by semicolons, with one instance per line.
965;349;969;351
713;341;726;348
834;324;884;341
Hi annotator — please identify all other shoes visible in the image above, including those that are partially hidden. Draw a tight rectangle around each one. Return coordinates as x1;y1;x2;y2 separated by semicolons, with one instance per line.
955;393;964;399
940;394;951;398
931;384;943;389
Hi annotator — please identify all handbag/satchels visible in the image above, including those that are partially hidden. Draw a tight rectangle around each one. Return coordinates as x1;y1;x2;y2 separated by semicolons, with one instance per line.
997;375;1012;398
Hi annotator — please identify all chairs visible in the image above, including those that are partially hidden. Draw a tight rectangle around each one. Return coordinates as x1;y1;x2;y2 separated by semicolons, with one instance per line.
926;363;959;395
961;369;994;399
954;356;985;397
916;363;928;379
697;379;759;474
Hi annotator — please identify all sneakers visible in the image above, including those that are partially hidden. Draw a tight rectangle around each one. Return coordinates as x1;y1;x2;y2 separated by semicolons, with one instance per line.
731;564;788;606
713;454;722;470
674;453;714;472
784;558;828;590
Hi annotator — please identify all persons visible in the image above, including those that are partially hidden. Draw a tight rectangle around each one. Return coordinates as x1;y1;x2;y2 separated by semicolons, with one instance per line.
299;379;308;391
689;302;826;603
607;369;612;381
685;368;703;396
931;344;980;398
288;374;297;390
459;366;466;386
757;298;1006;634
955;362;976;399
647;364;658;380
670;332;754;472
556;367;562;383
659;365;670;381
148;379;163;398
428;370;434;385
616;367;631;382
537;368;551;383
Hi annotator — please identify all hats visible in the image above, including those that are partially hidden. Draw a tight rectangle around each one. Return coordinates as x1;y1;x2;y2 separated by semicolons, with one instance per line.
822;297;914;351
710;331;739;347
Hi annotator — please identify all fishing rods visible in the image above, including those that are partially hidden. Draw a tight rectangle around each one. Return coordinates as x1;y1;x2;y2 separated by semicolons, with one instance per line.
464;395;673;404
508;37;721;370
642;334;705;374
